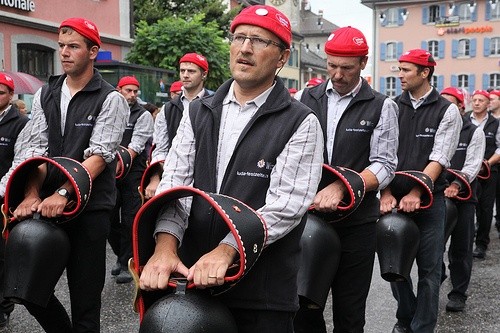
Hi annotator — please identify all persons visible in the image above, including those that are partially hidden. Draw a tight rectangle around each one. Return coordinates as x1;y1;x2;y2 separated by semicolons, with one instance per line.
294;25;399;333
440;87;500;310
0;17;130;333
108;53;209;283
379;49;463;333
139;5;323;333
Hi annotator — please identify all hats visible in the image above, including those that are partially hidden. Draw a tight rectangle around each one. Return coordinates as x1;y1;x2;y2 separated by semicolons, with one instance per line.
489;90;500;96
179;52;208;71
398;48;437;66
58;17;101;48
440;87;464;105
228;4;291;49
0;72;14;91
325;25;369;58
170;80;183;91
119;76;140;88
288;88;298;93
472;89;490;100
305;78;326;88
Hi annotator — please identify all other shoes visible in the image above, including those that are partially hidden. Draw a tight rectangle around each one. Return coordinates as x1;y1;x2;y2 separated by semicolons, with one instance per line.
116;271;133;283
473;246;486;258
446;299;466;311
111;263;121;275
392;320;409;333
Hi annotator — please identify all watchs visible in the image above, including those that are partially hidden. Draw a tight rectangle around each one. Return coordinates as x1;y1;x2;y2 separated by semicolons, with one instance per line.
55;188;70;197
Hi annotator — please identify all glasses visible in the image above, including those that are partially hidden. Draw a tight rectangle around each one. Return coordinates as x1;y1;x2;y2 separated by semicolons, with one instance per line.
231;33;283;52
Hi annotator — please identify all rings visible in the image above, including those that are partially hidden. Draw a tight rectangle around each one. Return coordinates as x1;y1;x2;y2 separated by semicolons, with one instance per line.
209;275;216;278
